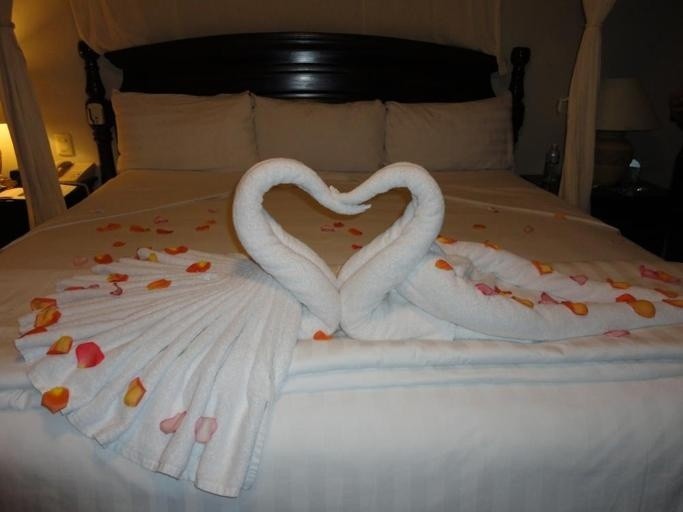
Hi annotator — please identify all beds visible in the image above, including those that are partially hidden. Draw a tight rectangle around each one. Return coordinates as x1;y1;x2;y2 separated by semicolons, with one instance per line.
0;28;683;512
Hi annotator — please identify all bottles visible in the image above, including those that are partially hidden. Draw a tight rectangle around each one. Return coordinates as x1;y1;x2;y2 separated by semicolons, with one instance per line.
544;144;560;182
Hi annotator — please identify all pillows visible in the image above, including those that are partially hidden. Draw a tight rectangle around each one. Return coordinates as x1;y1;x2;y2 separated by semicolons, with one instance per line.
249;92;386;173
387;95;515;172
106;88;259;173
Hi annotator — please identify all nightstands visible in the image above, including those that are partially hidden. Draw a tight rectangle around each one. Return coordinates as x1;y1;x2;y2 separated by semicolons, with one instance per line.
521;173;682;255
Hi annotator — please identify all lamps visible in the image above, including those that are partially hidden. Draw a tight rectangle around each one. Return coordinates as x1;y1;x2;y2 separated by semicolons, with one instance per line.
593;74;659;197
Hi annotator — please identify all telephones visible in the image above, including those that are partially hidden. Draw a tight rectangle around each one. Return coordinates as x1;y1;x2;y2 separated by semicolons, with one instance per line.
53;160;98;185
